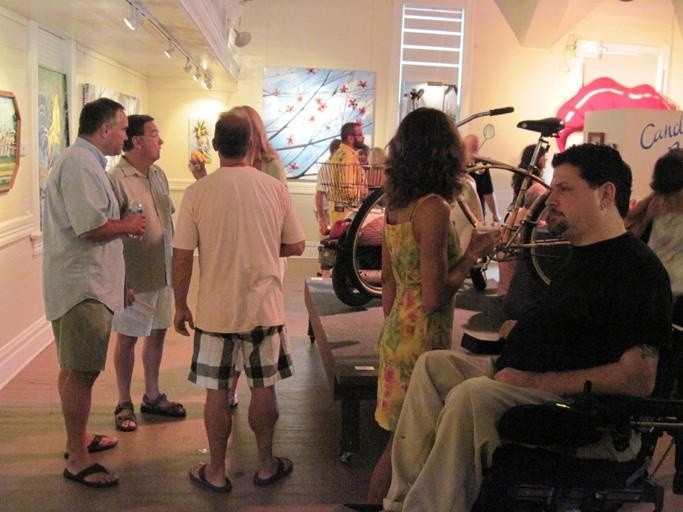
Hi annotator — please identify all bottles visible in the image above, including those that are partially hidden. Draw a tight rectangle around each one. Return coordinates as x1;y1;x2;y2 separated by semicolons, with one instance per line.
129;200;146;241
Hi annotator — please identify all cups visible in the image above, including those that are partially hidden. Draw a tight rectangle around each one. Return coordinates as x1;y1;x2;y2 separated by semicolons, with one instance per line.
476;222;501;232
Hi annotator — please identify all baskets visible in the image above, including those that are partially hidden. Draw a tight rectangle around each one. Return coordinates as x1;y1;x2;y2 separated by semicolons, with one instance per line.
316;159;387;209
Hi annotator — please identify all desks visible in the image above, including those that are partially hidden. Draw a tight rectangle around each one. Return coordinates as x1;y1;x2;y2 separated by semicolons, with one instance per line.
301;275;504;465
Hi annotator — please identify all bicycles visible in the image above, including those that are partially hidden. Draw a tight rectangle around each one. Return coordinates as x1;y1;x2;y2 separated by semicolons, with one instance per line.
346;81;562;307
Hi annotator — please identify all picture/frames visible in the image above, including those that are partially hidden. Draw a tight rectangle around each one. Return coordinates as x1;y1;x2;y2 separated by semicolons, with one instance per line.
38;63;69;231
0;90;21;194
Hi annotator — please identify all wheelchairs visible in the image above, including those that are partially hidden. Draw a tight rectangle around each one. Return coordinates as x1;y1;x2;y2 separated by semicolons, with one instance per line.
455;319;683;512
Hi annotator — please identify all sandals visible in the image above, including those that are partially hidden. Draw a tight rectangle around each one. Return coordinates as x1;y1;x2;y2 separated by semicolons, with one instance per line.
140;392;187;418
114;400;137;433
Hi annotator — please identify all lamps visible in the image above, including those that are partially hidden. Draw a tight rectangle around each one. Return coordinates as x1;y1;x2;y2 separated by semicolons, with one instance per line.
228;26;250;48
123;0;214;90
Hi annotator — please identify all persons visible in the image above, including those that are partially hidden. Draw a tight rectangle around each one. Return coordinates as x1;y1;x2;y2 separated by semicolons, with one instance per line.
367;108;505;512
188;102;288;410
450;135;551;294
41;98;147;489
625;146;683;328
106;113;186;432
169;115;305;495
382;142;674;511
315;107;388;278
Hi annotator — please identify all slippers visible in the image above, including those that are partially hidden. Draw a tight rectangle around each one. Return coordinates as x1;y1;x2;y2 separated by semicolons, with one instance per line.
190;463;231;494
63;461;119;489
63;431;118;458
252;456;293;485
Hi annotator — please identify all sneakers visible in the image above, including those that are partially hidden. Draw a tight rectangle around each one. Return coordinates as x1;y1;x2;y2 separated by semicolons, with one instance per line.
336;501;385;512
